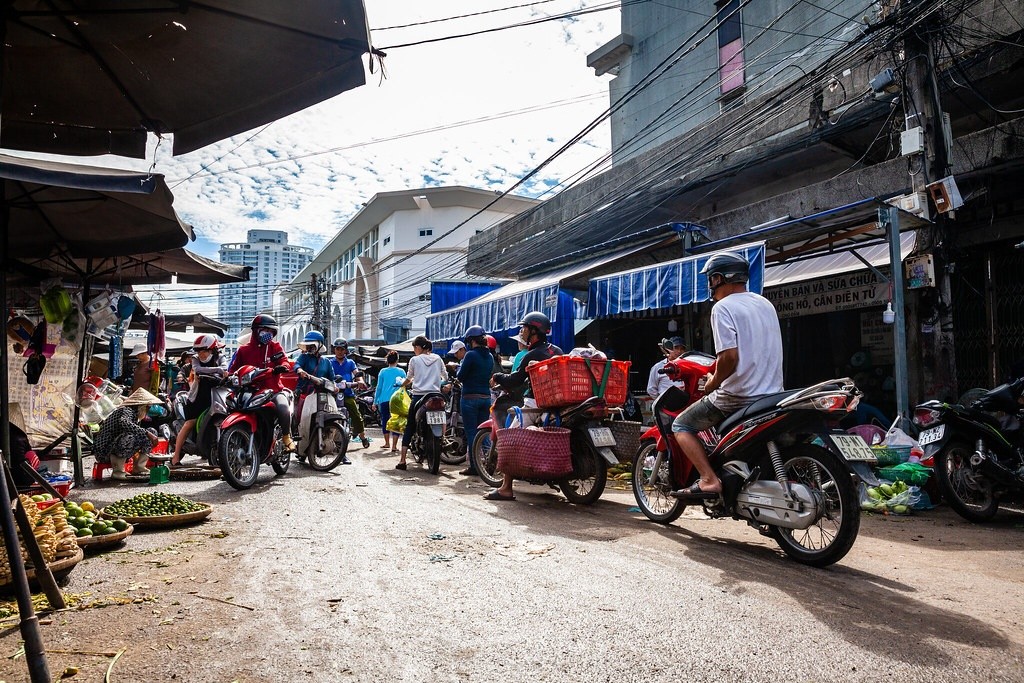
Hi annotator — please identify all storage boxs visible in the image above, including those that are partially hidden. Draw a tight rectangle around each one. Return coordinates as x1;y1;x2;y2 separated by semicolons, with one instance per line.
525;356;631;411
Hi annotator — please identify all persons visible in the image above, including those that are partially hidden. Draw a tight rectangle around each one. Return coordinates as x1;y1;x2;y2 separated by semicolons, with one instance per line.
647;336;686;399
95;386;166;479
373;311;567;501
170;314;370;467
128;343;160;394
0;421;38;490
671;252;783;497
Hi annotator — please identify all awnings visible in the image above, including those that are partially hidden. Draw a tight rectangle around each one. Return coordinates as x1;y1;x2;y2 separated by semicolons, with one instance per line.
127;314;228;339
425;234;682;343
587;198;936;320
347;333;425;369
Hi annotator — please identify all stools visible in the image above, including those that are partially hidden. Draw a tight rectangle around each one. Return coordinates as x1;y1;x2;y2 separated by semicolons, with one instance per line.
93;462;113;480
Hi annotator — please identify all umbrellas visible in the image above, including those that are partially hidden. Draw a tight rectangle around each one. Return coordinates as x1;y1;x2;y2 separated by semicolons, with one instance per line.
0;0;369;683
0;249;253;485
0;154;190;503
92;334;225;362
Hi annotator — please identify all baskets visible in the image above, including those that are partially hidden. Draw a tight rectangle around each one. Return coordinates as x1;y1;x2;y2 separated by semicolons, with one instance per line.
0;548;84;585
524;356;633;408
98;500;216;524
70;518;135;542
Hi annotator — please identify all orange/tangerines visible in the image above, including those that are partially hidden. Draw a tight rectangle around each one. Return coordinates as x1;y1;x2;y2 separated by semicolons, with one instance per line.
104;491;207;518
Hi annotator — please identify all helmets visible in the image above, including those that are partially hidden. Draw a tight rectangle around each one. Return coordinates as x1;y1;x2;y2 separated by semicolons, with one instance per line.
251;314;278;337
303;330;324;347
484;334;497;349
518;311;551;335
698;251;750;292
461;325;485;339
193;336;218;351
333;338;347;349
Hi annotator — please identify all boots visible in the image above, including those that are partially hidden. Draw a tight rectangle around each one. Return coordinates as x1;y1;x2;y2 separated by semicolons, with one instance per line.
111;454;132;479
132;452;152;475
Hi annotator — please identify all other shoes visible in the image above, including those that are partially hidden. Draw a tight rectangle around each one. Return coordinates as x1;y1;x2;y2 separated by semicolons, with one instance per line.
459;467;476;476
169;460;182;467
395;463;406;469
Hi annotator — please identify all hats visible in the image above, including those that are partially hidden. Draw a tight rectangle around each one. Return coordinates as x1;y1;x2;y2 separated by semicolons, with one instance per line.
296;340;327;353
509;334;527;346
119;387;165;406
446;341;465;354
129;343;148;357
667;335;689;347
217;342;226;350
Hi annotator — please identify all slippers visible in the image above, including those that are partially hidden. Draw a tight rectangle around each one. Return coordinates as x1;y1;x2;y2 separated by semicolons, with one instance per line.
669;483;720;497
380;445;390;448
283;441;296;453
359;435;370;448
483;488;517;499
392;449;399;453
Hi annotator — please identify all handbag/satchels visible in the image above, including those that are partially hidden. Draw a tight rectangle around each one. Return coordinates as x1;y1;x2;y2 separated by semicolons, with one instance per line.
386;385;413;436
850;420;935;513
6;280;134;387
603;408;644;458
495;406;573;481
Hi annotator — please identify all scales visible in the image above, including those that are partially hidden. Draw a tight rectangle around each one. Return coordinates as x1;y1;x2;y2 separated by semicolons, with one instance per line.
145;452;173;485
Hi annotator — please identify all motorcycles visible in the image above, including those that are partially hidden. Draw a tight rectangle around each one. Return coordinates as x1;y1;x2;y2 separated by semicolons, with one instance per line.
393;381;448;476
912;376;1024;524
215;353;290;491
294;371;382;472
470;382;619;504
157;372;233;467
441;388;468;465
631;352;882;571
